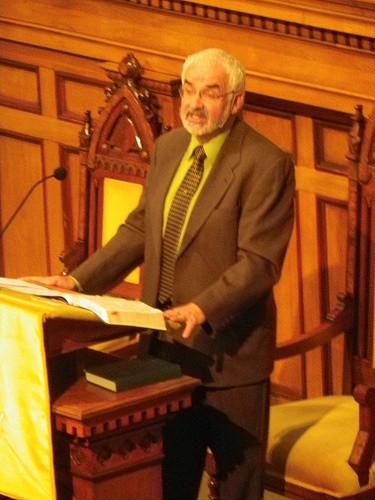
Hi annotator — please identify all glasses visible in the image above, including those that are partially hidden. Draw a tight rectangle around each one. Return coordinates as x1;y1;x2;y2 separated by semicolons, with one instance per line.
179;86;234;101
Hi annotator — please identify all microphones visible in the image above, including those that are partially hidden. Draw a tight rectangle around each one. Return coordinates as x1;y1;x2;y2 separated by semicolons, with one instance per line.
0;167;67;238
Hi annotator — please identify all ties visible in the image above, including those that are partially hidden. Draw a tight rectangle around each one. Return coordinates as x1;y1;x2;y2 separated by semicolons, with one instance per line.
157;146;206;304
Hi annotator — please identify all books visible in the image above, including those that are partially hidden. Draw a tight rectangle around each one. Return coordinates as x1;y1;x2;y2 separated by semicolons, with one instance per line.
83;355;182;393
1;277;167;331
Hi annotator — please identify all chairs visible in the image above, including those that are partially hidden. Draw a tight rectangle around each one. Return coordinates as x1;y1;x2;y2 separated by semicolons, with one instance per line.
202;102;375;500
59;51;172;303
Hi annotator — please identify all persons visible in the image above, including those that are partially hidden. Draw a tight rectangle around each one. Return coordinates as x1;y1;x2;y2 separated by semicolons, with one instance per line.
14;48;297;499
